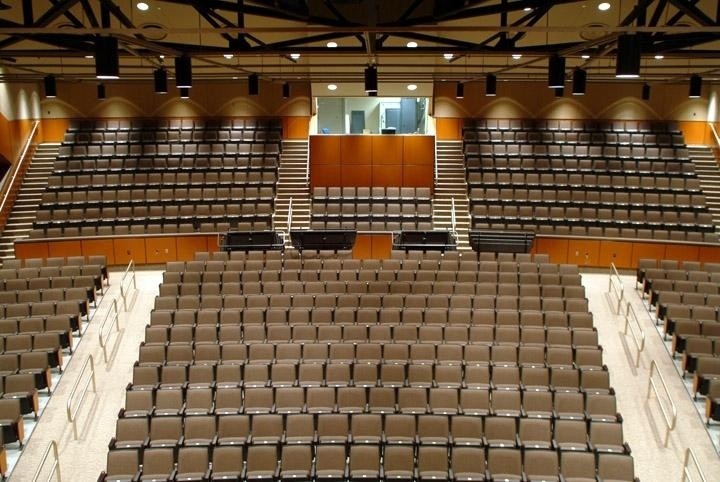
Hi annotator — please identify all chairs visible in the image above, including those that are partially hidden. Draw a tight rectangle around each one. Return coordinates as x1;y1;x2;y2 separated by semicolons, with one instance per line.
29;117;287;233
309;184;434;231
461;115;719;250
634;258;720;427
1;255;109;452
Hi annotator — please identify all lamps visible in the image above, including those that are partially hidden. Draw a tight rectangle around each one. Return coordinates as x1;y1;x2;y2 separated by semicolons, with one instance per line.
44;75;57;99
248;74;259;96
456;55;588;100
95;34;192;101
364;65;378;94
689;71;703;99
615;33;640;82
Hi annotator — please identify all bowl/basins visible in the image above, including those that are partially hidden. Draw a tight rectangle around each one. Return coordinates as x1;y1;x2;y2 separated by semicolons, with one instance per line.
321;127;329;134
